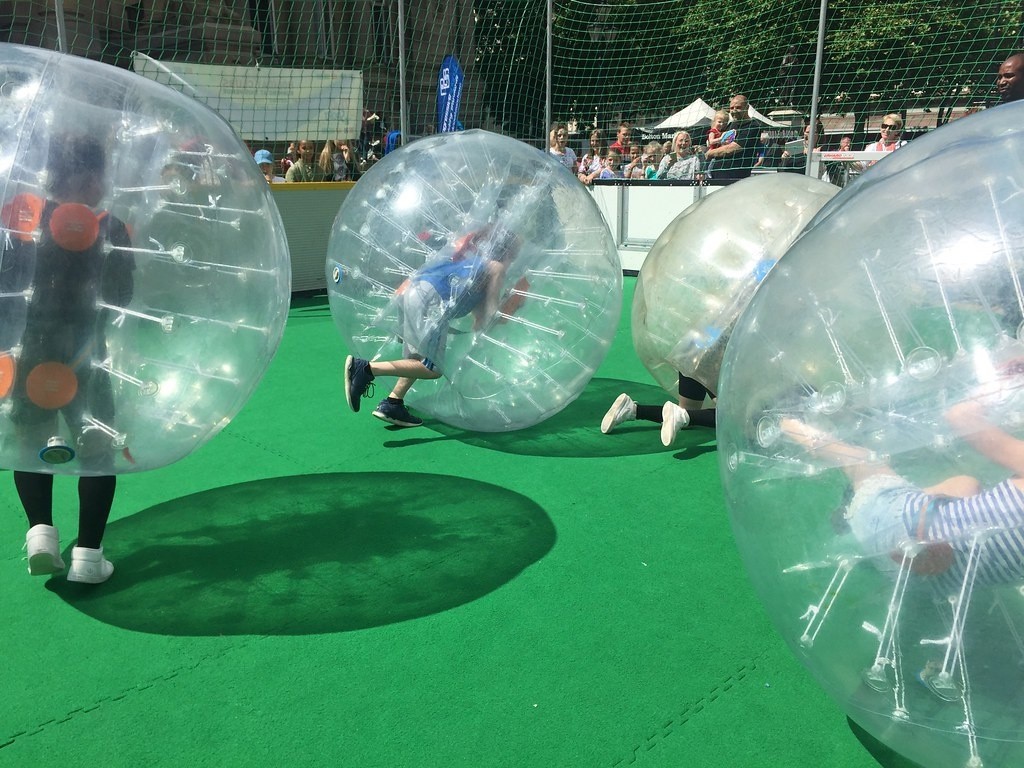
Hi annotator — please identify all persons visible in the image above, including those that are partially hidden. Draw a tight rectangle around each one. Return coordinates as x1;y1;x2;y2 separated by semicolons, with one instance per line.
541;95;907;189
285;108;403;183
157;137;247;190
345;223;531;427
254;149;285;182
1;130;138;584
779;401;1024;596
600;288;748;446
994;53;1024;103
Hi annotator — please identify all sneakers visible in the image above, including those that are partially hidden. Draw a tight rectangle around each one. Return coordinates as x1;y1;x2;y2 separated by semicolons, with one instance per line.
345;355;375;412
21;524;66;575
372;399;423;427
67;544;114;584
661;400;690;446
601;393;638;433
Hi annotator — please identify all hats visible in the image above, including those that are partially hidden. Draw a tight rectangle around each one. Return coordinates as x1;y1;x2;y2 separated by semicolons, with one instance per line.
254;149;273;165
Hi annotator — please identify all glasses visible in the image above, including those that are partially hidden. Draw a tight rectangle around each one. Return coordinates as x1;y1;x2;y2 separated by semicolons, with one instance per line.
881;124;896;130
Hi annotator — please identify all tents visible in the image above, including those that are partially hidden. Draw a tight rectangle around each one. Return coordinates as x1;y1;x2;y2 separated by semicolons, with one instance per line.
724;102;789;128
653;97;718;129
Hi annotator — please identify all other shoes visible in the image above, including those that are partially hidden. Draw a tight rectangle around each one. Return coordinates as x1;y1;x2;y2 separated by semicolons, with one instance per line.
833;483;854;534
755;385;818;427
